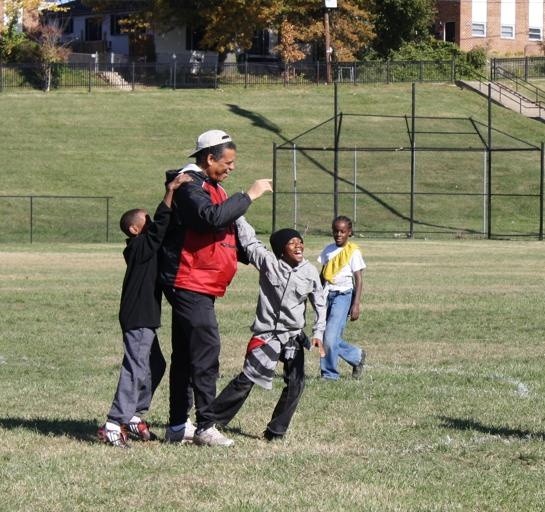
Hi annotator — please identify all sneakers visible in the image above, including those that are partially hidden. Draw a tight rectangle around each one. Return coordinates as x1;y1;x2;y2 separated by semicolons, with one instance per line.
98;416;158;449
165;418;235;447
353;350;367;377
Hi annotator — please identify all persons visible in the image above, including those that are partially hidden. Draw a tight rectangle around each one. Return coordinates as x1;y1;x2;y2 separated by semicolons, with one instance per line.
97;173;192;447
215;213;326;441
316;216;365;380
161;131;273;446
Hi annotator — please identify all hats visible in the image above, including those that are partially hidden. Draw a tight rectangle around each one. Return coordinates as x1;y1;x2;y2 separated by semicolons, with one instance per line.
188;130;232;158
269;228;302;257
